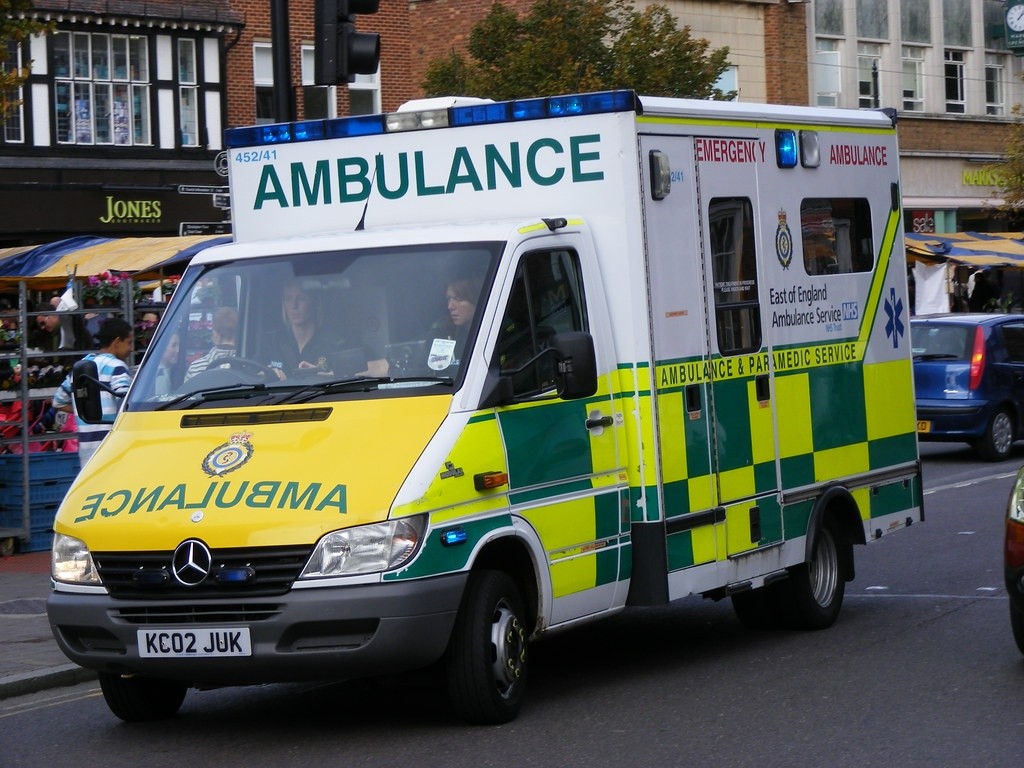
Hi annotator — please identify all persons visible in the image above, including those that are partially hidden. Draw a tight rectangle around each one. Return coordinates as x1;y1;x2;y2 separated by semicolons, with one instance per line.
0;272;350;473
419;265;535;405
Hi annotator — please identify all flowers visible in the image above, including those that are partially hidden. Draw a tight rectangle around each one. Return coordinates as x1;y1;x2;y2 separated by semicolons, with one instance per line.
83;271;142;305
187;317;216;343
164;276;181;296
134;320;161;340
193;277;218;302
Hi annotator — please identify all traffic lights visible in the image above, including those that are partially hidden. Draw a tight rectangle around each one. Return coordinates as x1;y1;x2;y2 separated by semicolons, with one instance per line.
314;1;379;87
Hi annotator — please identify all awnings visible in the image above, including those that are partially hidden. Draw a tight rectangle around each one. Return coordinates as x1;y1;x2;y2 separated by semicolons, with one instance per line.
0;231;237;543
904;231;1024;269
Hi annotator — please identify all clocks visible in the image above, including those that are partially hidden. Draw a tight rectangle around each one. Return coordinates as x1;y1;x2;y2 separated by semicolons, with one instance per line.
1007;4;1024;32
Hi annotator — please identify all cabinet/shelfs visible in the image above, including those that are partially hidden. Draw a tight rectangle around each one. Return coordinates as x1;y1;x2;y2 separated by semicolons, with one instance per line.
0;304;219;453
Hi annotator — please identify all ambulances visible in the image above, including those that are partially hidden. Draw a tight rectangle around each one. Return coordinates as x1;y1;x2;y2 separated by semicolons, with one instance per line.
48;89;925;726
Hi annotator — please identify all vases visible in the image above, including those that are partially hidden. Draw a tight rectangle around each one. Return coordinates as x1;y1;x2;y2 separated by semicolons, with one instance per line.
191;340;210;351
139;338;151;345
166;294;172;302
201;299;211;304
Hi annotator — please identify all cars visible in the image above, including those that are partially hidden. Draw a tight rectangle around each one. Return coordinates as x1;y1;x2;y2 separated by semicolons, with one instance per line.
1003;466;1024;655
910;313;1024;461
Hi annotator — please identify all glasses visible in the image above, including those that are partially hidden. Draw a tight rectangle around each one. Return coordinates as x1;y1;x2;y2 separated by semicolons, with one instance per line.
37;316;49;328
141;319;150;323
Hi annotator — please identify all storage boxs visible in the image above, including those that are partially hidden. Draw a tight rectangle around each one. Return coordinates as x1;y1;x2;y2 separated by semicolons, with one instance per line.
0;452;82;554
